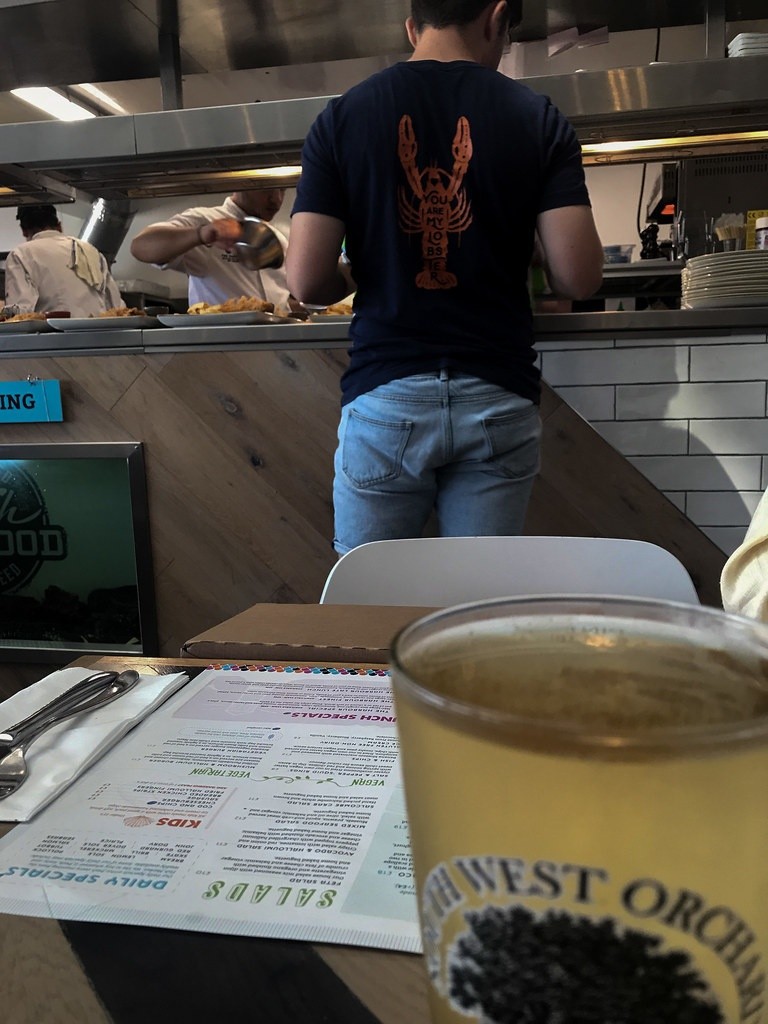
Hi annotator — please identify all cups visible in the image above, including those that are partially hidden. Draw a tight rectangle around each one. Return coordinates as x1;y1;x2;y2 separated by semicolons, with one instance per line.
389;594;768;1024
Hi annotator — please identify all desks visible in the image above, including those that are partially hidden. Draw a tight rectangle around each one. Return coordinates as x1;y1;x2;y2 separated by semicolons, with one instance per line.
180;603;441;665
0;653;429;1024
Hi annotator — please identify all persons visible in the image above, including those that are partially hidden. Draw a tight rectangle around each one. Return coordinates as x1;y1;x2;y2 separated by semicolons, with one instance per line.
131;189;302;314
4;205;127;317
285;0;606;560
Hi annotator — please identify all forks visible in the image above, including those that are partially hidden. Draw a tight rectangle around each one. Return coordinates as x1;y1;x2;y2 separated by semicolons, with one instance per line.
0;669;139;798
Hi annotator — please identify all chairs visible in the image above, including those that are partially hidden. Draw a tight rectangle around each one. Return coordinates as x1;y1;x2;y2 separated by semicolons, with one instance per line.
320;535;701;608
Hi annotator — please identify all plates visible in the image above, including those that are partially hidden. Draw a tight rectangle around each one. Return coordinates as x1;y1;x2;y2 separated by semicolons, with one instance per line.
681;249;768;309
47;315;160;333
157;310;265;328
727;32;768;58
310;313;355;322
0;319;53;333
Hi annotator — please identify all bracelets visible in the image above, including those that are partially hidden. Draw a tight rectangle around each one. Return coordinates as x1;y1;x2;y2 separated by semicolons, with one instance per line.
197;224;214;248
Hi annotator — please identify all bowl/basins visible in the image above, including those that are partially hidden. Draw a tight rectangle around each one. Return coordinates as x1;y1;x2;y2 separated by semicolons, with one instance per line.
230;220;284;271
